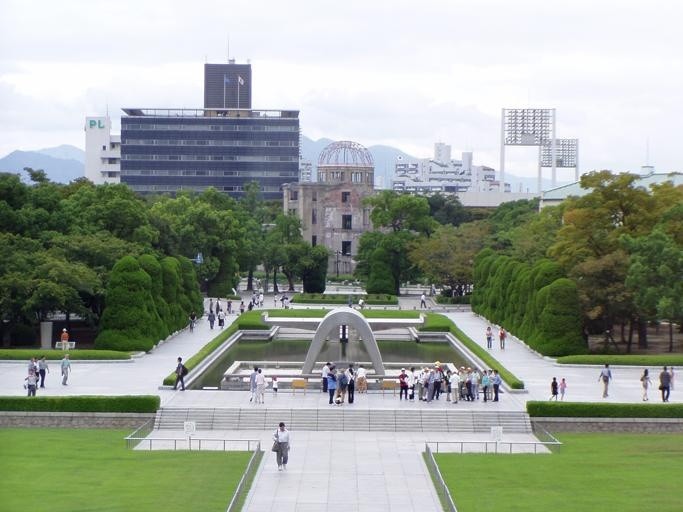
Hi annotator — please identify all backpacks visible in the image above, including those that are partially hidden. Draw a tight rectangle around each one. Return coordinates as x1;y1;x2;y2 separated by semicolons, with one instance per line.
182;365;189;377
349;374;354;386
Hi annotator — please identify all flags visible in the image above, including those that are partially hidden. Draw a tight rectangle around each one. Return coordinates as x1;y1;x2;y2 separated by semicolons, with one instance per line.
239;77;244;85
225;76;230;83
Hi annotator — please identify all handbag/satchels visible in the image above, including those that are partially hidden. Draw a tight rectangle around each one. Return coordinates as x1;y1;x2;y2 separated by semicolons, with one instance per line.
407;389;413;395
272;442;282;451
264;382;270;389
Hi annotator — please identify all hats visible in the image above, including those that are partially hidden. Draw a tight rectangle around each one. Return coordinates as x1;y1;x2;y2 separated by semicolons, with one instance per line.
63;328;67;331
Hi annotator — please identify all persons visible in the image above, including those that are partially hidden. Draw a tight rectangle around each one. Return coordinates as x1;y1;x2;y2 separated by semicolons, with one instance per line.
559;378;566;400
358;297;365;309
499;326;506;349
273;422;290;472
486;326;493;349
400;362;500;404
61;328;69;350
640;366;675;401
550;377;559;401
320;362;368;407
172;357;186;391
250;366;278;404
24;356;49;397
420;292;427;308
208;291;291;329
599;363;611;397
189;311;196;331
349;295;353;308
256;277;260;287
61;354;71;385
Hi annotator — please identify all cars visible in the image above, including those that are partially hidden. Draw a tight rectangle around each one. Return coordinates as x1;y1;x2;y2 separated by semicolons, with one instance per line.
659;319;676;326
